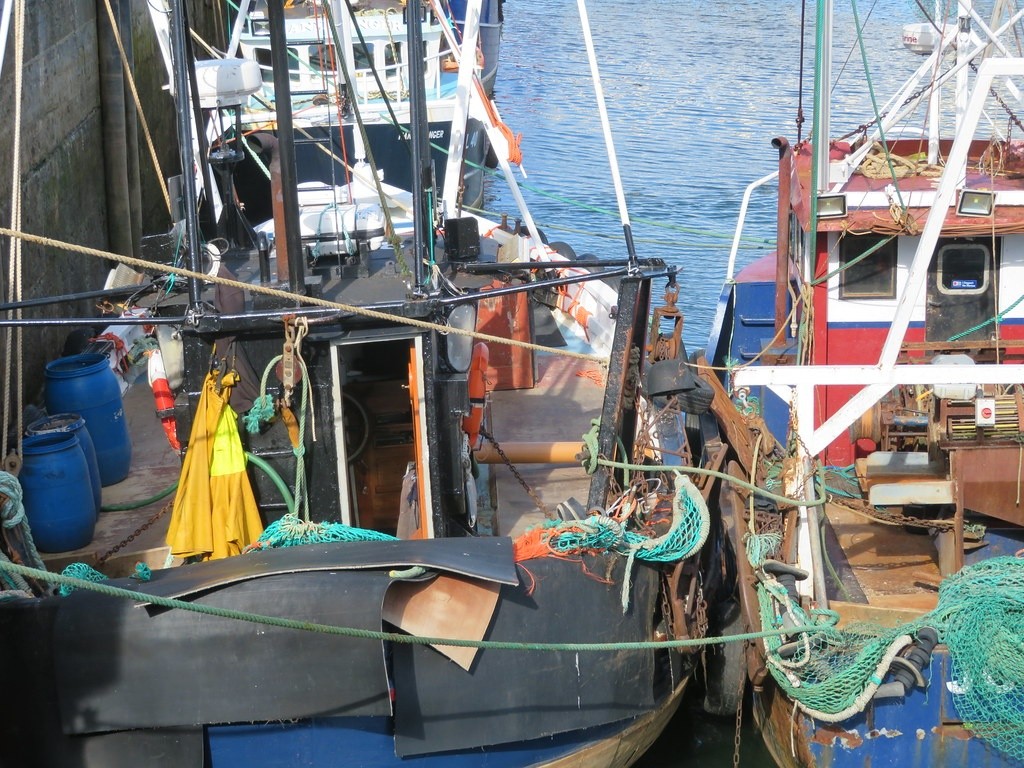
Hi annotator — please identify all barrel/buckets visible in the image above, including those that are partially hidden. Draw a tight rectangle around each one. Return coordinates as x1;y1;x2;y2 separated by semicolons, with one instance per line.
24;412;101;522
15;431;96;553
43;353;132;487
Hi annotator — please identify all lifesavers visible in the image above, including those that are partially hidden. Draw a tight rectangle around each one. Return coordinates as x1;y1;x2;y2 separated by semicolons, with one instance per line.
548;242;575;261
701;613;749;720
574;252;603;277
517;224;547;246
651;368;717;415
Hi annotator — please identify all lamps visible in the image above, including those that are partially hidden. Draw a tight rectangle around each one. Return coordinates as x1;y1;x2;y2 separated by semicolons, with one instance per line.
816;191;850;221
952;186;999;219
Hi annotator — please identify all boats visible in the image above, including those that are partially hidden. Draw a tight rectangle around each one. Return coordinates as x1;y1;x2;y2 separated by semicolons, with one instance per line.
0;0;730;767
691;0;1024;768
